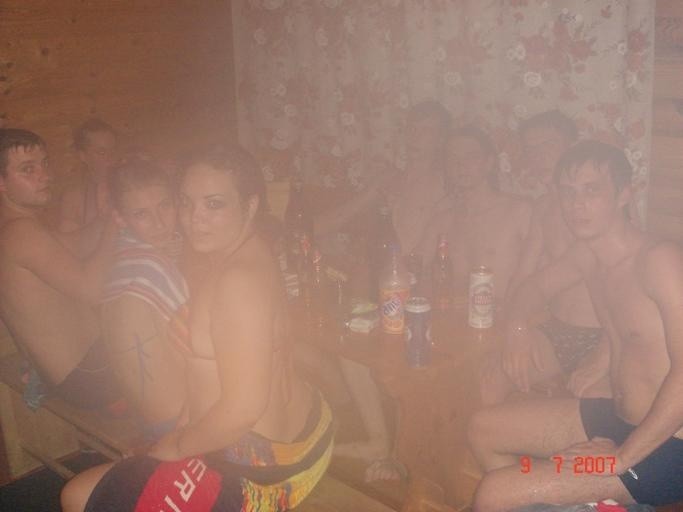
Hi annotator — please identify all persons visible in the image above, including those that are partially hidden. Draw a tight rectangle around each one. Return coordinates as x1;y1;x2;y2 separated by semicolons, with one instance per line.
0;99;682;511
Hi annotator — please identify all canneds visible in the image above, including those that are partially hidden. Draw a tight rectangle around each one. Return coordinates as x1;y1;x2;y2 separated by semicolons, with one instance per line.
466;267;494;329
404;296;433;366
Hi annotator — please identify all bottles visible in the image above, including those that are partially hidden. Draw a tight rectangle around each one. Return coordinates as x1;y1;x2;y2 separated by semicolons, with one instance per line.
291;206;452;334
